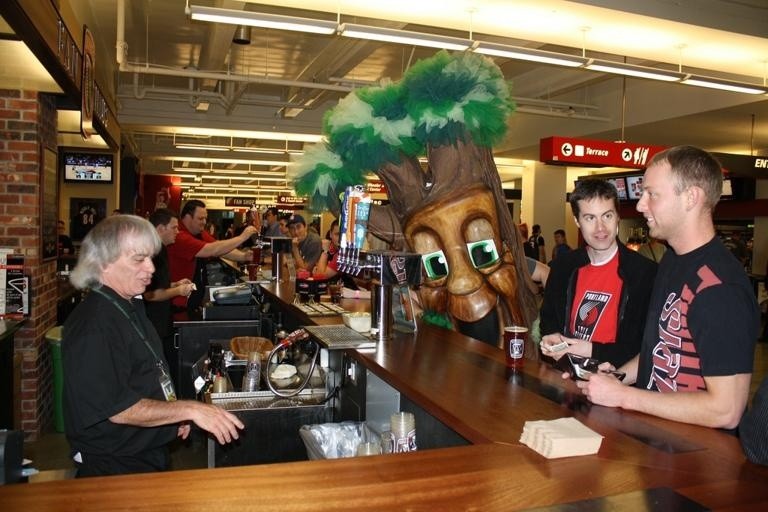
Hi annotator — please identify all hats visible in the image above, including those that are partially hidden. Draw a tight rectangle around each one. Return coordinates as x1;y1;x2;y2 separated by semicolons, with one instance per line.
285;214;306;228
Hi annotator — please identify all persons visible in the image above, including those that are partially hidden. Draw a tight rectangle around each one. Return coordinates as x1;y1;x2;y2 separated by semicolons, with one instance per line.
623;233;641;253
560;142;757;429
638;236;666;264
165;197;367;325
517;222;532;256
538;177;666;374
552;230;570;259
529;223;544;261
57;211;244;479
145;208;197;319
718;230;754;270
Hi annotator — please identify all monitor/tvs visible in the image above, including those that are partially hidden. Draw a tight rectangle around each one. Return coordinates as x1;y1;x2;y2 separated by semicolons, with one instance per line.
64;152;113;183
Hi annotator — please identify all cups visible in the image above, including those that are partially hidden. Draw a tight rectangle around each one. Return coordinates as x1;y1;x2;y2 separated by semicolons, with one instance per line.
504;327;529;367
356;410;418;456
242;353;261;391
248;247;262;281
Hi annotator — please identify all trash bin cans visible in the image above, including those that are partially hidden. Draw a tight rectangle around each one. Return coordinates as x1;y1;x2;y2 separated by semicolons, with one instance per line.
45;326;65;434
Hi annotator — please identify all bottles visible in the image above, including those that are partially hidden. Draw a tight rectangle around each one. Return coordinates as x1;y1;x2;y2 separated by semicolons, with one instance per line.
204;341;228;393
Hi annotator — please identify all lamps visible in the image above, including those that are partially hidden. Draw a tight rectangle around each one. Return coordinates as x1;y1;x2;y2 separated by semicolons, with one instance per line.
171;133;306;193
